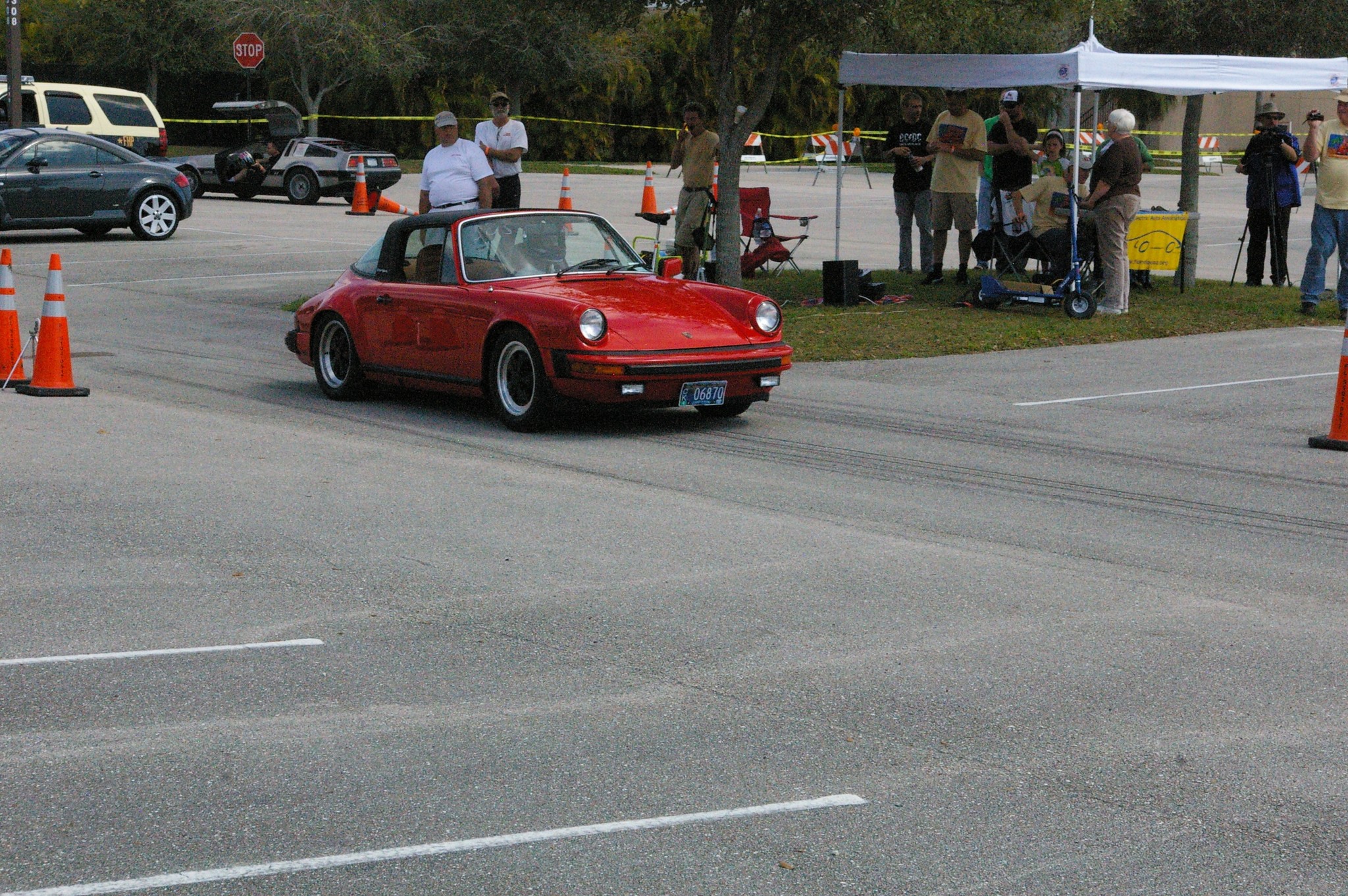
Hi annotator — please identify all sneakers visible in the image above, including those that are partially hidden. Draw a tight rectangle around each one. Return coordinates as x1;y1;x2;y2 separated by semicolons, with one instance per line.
924;270;944;284
956;273;970;286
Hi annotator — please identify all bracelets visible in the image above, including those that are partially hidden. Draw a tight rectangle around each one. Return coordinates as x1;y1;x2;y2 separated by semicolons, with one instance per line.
951;146;955;154
1018;213;1022;216
485;146;489;155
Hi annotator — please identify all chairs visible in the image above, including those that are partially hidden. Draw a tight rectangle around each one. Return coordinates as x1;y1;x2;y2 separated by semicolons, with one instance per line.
416;244;458;284
991;185;1060;290
739;187;819;279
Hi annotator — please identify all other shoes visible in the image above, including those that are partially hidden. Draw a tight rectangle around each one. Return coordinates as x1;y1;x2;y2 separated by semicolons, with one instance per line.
1122;309;1128;314
1302;302;1316;315
1143;282;1155;292
1338;308;1348;319
974;264;988;270
1129;281;1145;290
1096;305;1122;315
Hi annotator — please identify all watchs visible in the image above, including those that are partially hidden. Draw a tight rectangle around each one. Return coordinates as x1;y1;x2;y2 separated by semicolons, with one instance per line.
1279;139;1284;145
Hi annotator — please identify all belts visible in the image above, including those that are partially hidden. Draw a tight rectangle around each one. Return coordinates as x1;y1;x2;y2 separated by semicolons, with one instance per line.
684;184;713;192
432;197;480;209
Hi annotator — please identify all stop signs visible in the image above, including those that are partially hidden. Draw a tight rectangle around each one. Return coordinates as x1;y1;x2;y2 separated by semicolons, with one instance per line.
232;32;265;69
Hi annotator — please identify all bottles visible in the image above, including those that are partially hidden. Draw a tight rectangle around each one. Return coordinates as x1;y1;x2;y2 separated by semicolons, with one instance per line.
909;155;923;172
753;208;762;237
710;245;716;260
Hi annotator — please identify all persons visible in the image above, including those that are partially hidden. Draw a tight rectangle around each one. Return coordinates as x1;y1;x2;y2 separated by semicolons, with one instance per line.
671;101;722;279
1233;102;1302;287
227;141;283;183
886;88;1154;314
474;92;528;263
419;111;493;260
1299;88;1348;319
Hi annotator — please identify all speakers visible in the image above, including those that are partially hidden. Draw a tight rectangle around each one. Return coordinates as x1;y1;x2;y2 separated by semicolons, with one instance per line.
823;260;857;306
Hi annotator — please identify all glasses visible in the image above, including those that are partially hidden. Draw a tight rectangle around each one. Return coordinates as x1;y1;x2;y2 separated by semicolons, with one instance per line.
491;101;509;107
1106;121;1112;125
1263;114;1278;120
1004;103;1019;109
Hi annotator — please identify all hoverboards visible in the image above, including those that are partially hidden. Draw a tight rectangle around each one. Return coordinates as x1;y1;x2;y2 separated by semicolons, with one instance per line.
972;182;1098;320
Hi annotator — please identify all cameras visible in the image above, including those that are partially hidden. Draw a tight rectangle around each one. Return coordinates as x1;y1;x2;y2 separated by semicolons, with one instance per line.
1309;113;1324;120
1256;124;1287;136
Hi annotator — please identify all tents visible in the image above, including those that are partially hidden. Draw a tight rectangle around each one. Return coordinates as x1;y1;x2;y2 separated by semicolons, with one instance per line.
834;35;1348;288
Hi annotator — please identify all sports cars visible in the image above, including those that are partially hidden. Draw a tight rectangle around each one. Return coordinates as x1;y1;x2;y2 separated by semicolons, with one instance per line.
167;99;401;205
284;208;796;432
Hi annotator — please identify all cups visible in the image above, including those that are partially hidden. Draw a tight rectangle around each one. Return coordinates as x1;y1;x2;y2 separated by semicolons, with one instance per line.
264;154;268;160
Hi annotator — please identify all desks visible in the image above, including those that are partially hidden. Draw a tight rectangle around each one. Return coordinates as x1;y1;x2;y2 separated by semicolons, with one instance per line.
1057;208;1201;299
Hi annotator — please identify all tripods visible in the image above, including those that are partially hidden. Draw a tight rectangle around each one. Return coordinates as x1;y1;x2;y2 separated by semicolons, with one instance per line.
1230;138;1292;287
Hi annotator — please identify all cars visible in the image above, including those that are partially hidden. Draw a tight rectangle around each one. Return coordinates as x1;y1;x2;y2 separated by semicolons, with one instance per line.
0;128;194;241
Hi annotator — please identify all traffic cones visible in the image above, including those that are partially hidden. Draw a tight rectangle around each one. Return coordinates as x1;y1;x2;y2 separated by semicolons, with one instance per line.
346;155;375;216
661;205;680;216
14;254;91;397
556;168;580;235
1308;310;1348;452
0;248;31;387
636;161;663;216
376;195;420;215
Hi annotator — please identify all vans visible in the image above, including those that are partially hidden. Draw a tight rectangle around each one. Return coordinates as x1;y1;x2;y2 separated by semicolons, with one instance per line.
0;75;169;160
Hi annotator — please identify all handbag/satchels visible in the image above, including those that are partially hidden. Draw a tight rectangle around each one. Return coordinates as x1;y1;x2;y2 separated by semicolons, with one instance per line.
691;226;715;250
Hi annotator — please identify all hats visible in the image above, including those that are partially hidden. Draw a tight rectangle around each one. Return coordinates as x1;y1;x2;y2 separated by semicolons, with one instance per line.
489;92;510;104
435;111;457;127
1067;154;1093;169
1003;90;1021;103
1254;102;1285;123
1334;88;1348;102
